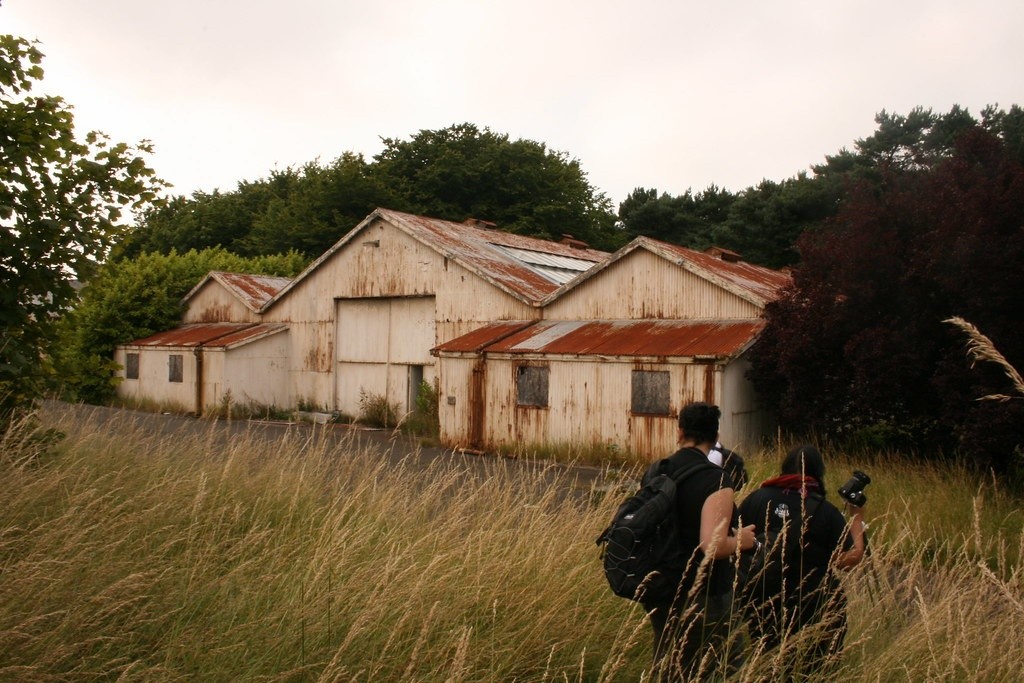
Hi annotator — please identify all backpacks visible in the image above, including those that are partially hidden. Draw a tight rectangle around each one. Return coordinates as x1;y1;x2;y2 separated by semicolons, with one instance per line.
711;444;744;492
599;456;720;602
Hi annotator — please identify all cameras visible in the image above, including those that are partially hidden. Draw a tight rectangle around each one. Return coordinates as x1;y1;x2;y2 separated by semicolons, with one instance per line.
838;470;871;508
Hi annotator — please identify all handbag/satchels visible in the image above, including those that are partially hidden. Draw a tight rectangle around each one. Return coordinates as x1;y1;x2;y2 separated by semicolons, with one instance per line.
742;495;820;597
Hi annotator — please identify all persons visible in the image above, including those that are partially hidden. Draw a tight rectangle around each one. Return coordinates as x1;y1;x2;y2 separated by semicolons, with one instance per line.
637;402;755;683
737;445;865;683
